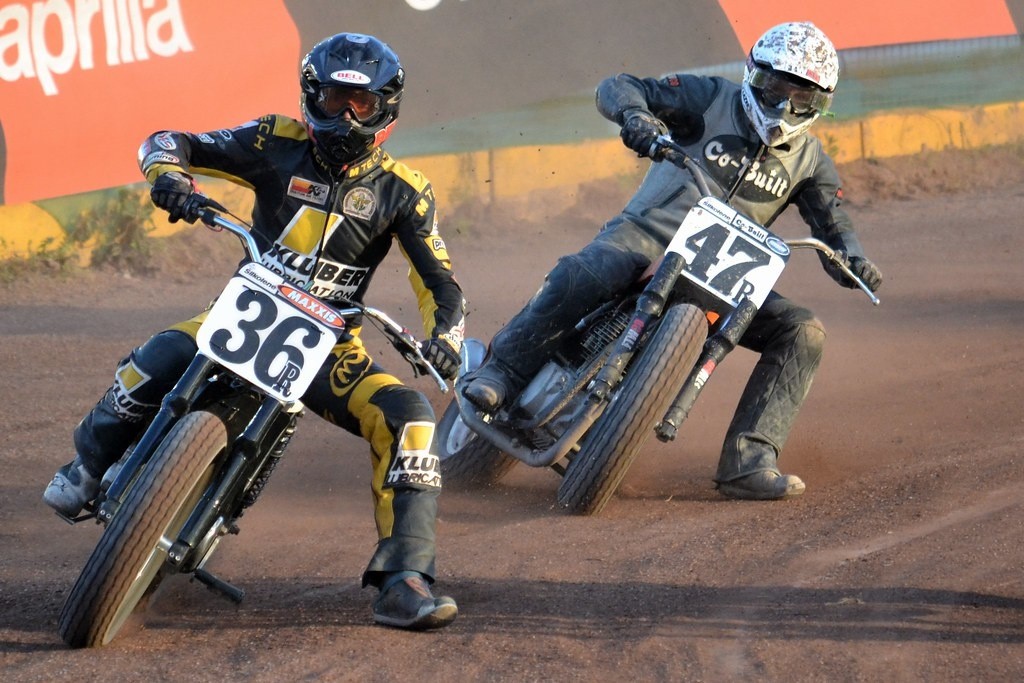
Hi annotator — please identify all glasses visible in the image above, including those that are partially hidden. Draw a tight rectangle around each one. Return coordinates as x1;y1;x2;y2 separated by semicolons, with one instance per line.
311;84;388;123
748;69;822;116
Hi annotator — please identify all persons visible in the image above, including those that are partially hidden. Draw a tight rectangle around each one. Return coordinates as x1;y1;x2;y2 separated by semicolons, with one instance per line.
466;20;883;501
44;32;468;632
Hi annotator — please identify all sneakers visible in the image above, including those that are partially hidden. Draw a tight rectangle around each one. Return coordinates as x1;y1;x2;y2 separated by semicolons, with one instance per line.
373;573;458;629
42;454;101;518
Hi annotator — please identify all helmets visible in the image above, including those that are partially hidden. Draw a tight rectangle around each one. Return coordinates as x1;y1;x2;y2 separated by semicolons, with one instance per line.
300;31;405;167
741;21;840;148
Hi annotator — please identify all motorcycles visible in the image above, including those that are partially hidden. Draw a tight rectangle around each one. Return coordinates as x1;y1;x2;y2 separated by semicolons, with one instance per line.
50;194;462;651
433;131;882;518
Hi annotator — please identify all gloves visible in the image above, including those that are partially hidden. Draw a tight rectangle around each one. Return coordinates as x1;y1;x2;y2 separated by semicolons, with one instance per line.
839;257;883;293
616;108;671;163
151;170;196;223
412;337;463;381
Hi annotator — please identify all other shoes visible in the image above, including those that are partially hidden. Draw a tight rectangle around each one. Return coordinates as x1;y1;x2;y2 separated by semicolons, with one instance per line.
461;346;519;411
720;471;806;499
